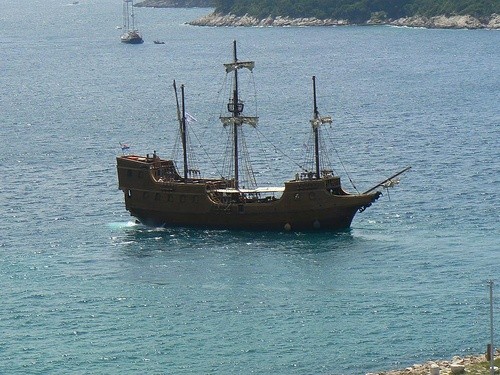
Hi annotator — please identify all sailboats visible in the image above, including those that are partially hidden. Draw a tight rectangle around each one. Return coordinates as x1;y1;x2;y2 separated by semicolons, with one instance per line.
120;0;144;44
116;39;410;231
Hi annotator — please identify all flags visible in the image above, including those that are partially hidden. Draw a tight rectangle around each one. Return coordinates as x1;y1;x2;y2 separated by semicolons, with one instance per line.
120;143;130;150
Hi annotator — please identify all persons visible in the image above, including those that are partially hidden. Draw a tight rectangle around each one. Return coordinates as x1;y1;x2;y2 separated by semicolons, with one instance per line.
158;176;164;183
146;154;150;163
152;150;156;162
